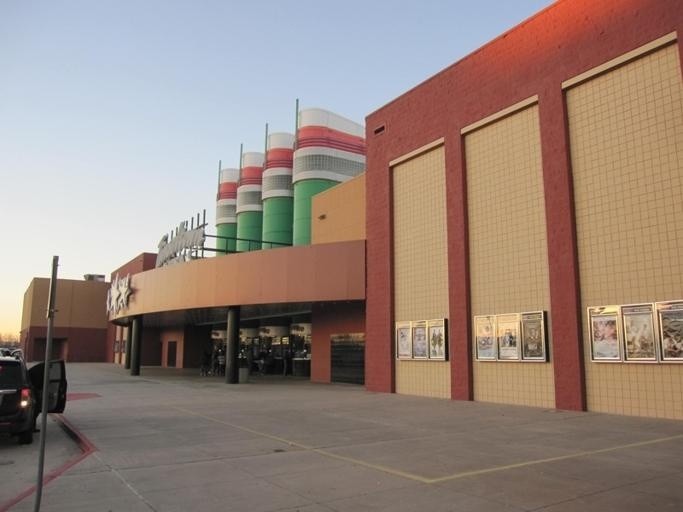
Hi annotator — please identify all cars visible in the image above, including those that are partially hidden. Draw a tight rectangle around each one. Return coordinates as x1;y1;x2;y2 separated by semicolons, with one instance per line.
0;347;24;359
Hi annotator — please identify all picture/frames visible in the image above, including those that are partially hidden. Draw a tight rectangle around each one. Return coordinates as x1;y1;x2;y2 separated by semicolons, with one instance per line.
394;318;446;361
586;299;683;364
473;310;547;363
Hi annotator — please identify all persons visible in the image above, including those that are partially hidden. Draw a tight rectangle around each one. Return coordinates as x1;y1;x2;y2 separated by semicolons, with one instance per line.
432;329;443;349
594;319;619;358
199;344;253;376
526;328;542;357
664;335;678;356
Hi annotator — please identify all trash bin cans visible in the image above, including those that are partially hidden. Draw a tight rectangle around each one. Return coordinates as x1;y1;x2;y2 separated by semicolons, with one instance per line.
238;368;249;384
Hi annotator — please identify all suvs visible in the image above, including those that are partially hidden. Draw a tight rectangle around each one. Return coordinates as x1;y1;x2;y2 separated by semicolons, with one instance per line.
0;358;70;451
